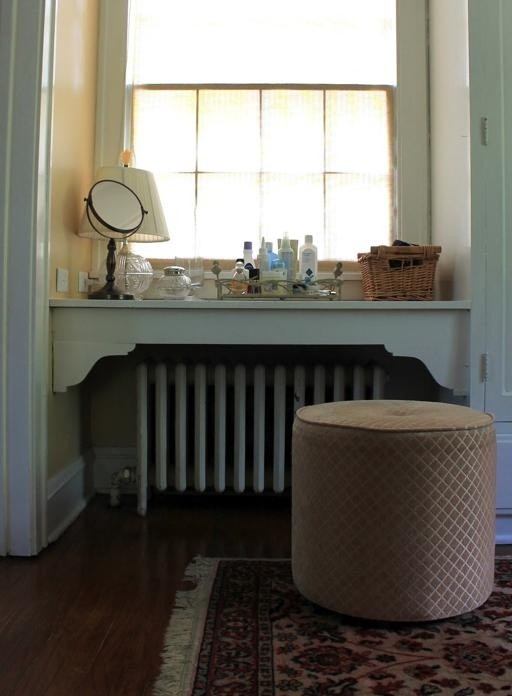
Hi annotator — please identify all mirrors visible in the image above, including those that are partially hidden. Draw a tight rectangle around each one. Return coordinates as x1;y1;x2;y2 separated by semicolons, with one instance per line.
80;177;148;299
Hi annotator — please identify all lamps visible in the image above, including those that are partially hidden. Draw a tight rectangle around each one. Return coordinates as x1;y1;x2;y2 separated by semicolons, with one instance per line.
76;164;170;298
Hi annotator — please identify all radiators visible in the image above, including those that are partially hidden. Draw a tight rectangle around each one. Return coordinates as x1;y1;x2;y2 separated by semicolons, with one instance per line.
110;357;392;515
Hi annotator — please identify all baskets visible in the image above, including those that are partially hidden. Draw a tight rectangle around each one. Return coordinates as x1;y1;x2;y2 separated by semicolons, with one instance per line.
358;245;441;301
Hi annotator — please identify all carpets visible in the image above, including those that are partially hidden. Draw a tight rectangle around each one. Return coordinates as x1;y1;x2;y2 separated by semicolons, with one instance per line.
153;553;512;695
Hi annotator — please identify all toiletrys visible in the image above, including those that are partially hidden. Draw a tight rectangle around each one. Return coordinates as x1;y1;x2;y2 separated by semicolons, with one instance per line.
226;233;319;298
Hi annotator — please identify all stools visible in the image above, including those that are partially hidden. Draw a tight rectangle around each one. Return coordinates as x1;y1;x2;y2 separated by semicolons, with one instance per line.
288;398;496;623
